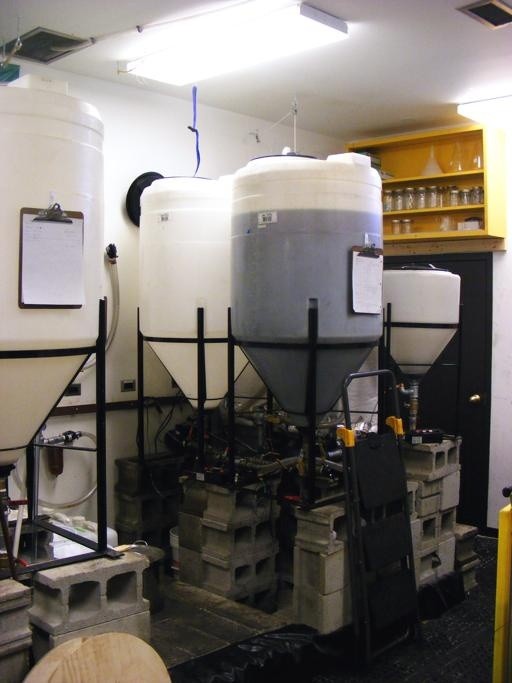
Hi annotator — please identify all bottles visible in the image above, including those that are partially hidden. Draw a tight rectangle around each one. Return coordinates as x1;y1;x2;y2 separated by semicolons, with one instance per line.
422;140;483;177
382;185;485;235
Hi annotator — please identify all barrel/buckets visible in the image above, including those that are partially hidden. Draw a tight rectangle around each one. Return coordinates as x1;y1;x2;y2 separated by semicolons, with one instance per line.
137;175;247;409
231;148;383;421
382;265;461;374
0;75;105;464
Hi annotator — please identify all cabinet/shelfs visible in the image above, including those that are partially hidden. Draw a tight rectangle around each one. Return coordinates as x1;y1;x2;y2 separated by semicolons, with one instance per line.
346;124;506;242
